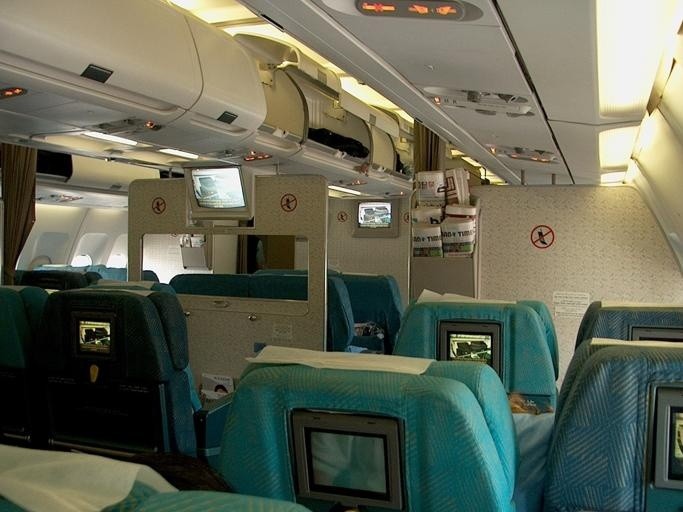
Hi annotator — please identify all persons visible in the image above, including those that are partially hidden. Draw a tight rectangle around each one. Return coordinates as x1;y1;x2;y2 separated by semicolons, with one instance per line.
505;393;557;512
250;236;266;274
123;449;238;492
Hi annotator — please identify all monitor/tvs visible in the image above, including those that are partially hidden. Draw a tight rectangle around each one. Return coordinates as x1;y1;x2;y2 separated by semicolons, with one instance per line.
34;281;64;294
242;234;295;274
438;320;502;379
78;318;114;355
353;201;400;237
182;162;256;221
654;387;683;491
632;328;682;342
289;408;403;508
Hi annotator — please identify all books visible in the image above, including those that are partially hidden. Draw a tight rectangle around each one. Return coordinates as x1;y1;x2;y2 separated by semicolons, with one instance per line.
199;373;237;402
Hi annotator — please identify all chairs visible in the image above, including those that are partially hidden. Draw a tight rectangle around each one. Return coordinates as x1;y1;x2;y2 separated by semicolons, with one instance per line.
2;266;683;512
